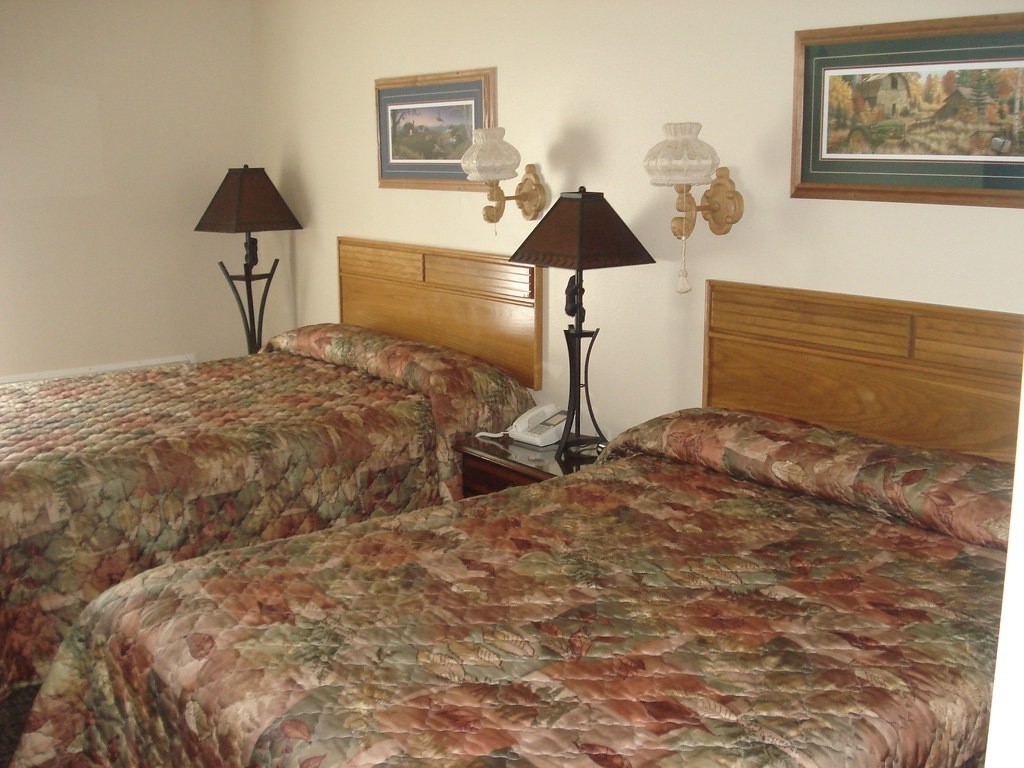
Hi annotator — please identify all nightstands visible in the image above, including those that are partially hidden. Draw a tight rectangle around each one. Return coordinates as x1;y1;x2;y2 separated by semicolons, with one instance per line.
454;432;609;498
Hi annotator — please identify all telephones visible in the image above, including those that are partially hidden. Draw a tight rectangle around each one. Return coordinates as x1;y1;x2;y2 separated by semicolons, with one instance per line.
507;402;568;447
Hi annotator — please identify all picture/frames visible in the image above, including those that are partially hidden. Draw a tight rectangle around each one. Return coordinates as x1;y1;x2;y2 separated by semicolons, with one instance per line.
790;11;1024;209
374;66;498;193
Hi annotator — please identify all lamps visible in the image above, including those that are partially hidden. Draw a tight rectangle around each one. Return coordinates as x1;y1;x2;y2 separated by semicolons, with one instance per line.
508;185;657;461
642;121;744;294
460;127;546;237
194;163;303;354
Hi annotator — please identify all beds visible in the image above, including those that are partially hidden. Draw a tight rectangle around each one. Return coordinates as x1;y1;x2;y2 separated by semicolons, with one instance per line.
7;280;1024;768
0;236;543;768
